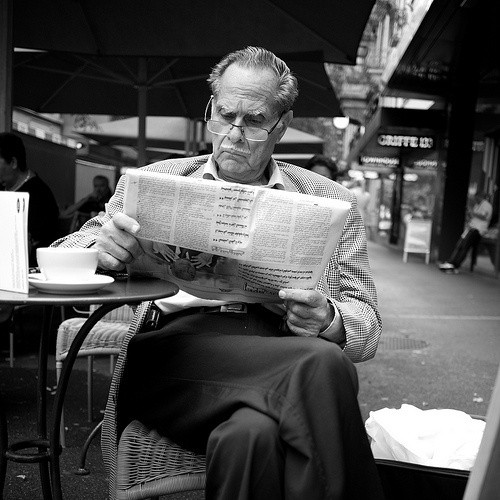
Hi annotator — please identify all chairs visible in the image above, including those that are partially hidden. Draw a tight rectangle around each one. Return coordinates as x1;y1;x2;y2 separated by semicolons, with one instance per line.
100;299;207;500
56;304;135;448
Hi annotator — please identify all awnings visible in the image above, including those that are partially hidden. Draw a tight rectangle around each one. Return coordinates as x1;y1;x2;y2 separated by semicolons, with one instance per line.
346;106;447;168
384;0;500;100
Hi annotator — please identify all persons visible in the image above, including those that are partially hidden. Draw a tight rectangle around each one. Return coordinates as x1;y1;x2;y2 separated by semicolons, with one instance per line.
48;46;381;500
306;155;338;182
0;131;59;269
440;191;493;269
79;175;111;227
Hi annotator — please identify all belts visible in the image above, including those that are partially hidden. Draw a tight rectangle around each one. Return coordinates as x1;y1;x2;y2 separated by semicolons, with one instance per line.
159;303;284;321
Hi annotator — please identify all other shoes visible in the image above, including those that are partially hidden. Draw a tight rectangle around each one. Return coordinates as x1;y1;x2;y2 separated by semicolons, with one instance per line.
439;262;459;274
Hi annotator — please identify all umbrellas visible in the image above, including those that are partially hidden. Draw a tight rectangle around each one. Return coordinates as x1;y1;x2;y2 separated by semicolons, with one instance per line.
0;0;380;170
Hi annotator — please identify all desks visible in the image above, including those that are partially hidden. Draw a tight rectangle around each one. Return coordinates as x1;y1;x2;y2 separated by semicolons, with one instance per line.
0;278;179;500
403;218;432;265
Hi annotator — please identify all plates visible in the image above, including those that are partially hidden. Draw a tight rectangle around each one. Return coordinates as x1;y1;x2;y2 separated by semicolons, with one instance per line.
27;272;114;295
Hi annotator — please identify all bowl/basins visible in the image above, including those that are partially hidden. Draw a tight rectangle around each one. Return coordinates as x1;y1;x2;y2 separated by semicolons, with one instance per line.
36;247;99;282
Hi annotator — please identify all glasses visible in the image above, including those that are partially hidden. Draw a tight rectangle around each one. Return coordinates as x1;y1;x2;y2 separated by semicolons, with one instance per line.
204;93;286;143
